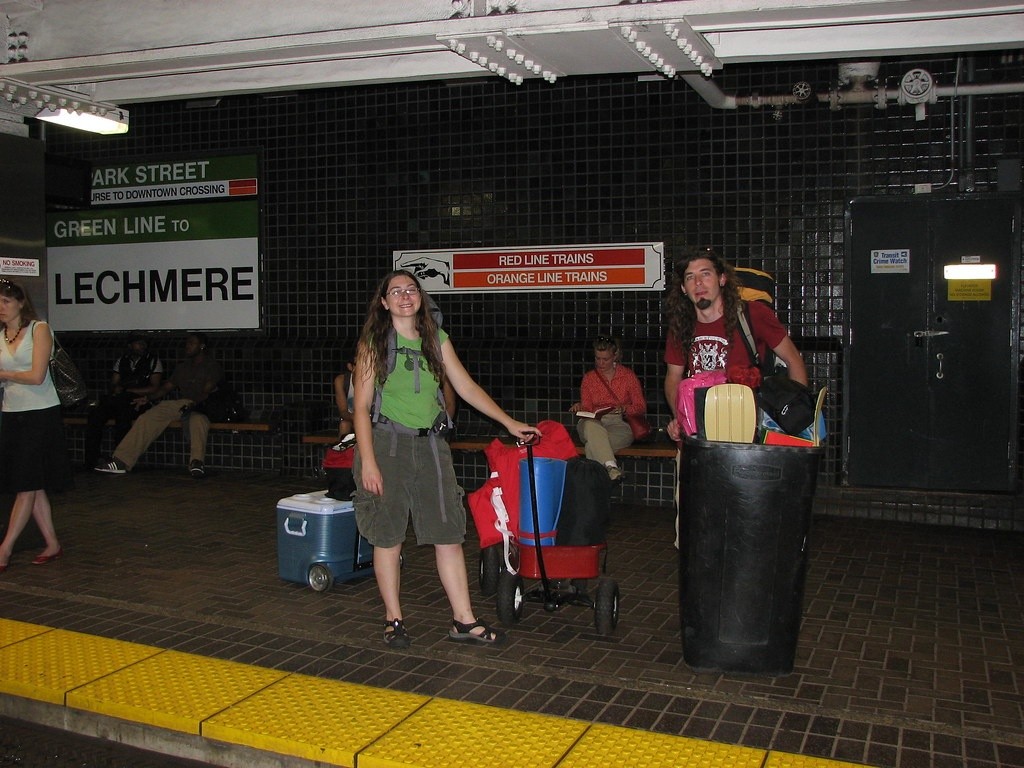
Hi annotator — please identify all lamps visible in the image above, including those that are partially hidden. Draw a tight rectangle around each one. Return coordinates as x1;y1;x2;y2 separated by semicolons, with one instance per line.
34;100;129;135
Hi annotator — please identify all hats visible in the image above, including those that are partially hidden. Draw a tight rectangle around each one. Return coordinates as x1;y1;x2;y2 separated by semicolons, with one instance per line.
126;329;147;343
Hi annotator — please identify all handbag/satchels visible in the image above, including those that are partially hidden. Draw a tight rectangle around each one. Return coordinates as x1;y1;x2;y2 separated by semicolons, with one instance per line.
625;412;652;443
323;432;367;502
196;388;251;426
757;372;815;433
29;321;87;407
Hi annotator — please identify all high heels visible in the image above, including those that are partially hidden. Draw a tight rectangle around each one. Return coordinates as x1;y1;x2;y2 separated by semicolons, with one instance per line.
32;546;64;564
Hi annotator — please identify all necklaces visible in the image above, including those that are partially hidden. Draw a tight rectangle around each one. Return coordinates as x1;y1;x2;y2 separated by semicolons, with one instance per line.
5;324;22;343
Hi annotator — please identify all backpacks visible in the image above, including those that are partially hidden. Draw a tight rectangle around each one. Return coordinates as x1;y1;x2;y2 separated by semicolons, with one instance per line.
731;266;777;380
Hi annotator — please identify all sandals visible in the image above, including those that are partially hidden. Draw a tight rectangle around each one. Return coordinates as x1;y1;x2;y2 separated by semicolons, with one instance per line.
448;617;507;642
383;617;410;648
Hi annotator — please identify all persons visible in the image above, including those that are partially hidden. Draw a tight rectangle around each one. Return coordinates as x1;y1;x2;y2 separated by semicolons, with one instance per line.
94;332;224;477
335;346;455;441
74;331;163;473
353;270;541;647
664;247;806;550
569;337;647;482
0;279;64;571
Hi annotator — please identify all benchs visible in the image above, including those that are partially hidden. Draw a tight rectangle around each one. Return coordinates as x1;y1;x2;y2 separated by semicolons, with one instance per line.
60;416;270;433
304;428;678;457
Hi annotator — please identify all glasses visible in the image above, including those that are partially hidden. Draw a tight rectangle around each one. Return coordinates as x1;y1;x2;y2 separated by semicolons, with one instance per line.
700;247;712;253
596;336;617;348
386;287;420;296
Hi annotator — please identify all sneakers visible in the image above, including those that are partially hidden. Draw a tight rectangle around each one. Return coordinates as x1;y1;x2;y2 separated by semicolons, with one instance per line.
190;461;208;480
94;458;126;474
606;464;625;484
